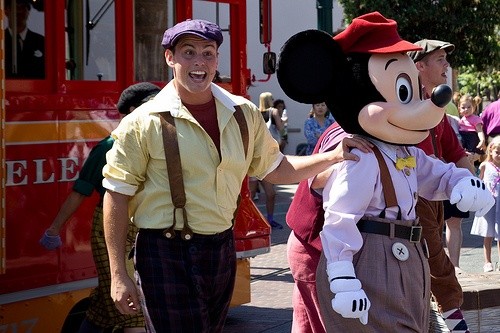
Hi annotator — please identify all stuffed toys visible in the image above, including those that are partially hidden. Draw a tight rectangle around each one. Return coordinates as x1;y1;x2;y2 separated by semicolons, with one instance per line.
276;12;495;333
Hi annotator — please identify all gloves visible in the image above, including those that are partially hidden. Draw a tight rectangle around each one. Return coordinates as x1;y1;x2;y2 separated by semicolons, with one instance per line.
39;233;61;250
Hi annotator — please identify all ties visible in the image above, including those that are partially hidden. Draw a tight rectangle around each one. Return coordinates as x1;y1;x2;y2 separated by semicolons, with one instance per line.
16;33;21;52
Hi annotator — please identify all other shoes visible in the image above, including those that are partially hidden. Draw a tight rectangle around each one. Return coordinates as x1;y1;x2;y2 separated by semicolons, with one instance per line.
268;221;282;229
455;267;468;277
484;263;500;272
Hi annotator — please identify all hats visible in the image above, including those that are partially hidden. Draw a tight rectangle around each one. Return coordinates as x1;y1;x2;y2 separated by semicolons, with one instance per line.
117;82;161;114
407;39;455;64
161;19;223;48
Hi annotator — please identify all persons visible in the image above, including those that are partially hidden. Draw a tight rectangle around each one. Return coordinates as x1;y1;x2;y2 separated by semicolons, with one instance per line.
285;122;357;333
302;102;336;156
438;64;500;272
401;39;478;333
257;92;288;153
41;82;162;333
102;20;373;333
248;175;282;230
4;0;45;80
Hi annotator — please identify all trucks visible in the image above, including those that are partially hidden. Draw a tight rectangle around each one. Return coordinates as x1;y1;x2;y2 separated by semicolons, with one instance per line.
0;0;277;333
